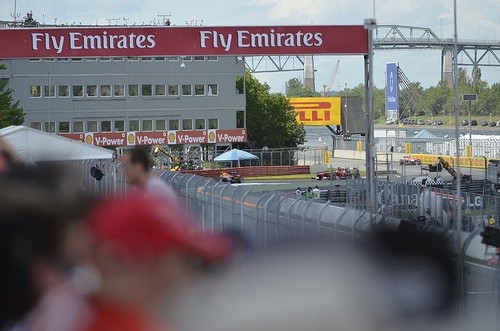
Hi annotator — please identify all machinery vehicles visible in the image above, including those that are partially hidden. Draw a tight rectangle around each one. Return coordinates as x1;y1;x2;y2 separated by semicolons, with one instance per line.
421;156;472;182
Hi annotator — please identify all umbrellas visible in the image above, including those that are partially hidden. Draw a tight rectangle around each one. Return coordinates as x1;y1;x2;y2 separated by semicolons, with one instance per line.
213;148;260;168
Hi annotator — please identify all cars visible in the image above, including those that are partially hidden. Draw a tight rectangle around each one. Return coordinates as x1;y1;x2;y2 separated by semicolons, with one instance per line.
462;120;468;125
481;121;488;126
435;120;444;125
399;118;434;125
491;121;500;127
399;156;422;166
471;120;478;126
311;167;358;180
220;171;245;183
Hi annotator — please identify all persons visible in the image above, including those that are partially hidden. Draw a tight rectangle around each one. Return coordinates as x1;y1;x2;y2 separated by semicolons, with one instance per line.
0;136;500;331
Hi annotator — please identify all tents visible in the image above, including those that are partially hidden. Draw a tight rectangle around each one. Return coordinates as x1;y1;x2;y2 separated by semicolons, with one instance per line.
0;125;116;197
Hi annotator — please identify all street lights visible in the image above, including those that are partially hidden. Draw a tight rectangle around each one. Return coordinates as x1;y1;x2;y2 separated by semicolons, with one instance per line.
462;94;476;157
